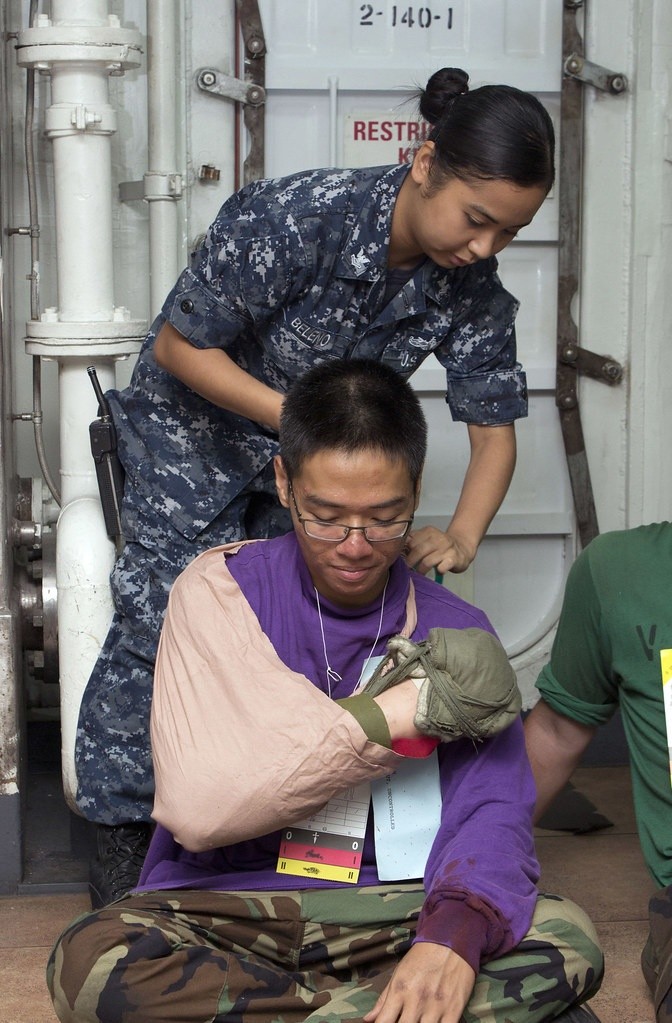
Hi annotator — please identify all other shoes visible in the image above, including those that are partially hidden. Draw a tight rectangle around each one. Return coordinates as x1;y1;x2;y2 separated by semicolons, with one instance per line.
89;824;151;910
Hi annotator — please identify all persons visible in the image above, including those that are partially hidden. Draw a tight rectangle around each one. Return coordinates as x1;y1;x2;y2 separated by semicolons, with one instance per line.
77;69;555;911
518;520;672;1023
44;356;607;1022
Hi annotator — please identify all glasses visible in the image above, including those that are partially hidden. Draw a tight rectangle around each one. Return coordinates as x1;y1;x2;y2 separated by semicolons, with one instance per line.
287;472;417;542
